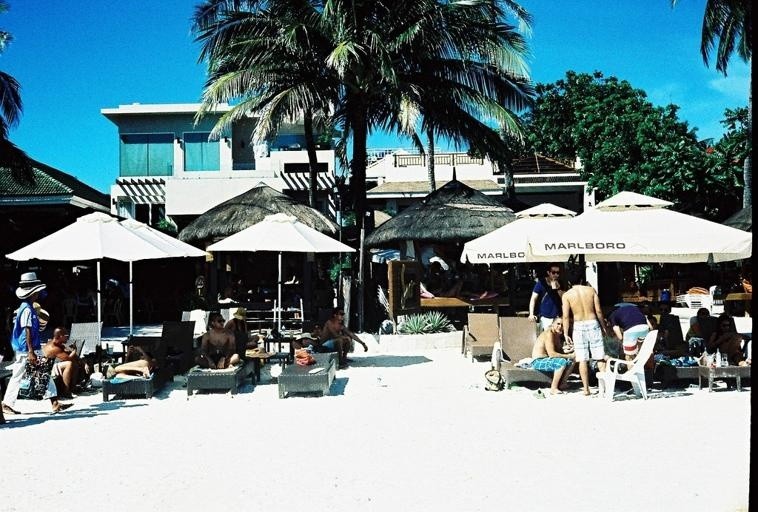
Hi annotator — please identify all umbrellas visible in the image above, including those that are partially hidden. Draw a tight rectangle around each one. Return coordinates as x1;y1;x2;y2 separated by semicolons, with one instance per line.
460;191;754;264
5;211;186;348
119;215;206;337
203;211;356;332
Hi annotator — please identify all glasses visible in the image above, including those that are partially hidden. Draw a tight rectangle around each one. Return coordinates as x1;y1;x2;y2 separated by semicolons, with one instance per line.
218;319;225;323
552;271;559;275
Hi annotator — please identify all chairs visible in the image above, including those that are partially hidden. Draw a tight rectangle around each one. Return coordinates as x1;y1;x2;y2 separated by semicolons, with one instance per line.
454;286;752;401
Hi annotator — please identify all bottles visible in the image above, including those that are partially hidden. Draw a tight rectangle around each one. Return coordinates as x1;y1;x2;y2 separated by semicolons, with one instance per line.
661;288;671;300
702;347;722;368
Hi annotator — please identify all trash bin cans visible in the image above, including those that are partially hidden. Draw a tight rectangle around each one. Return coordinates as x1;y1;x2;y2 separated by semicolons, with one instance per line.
661;291;670;301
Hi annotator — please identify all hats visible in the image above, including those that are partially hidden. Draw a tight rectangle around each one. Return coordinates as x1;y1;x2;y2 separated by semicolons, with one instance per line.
296;333;317;340
15;272;47;299
233;307;247;321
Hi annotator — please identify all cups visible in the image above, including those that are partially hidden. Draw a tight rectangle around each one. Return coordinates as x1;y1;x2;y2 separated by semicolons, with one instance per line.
722;353;727;366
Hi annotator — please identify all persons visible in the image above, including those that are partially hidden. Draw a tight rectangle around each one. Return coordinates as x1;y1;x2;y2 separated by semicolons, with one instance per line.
421;256;508;301
316;306;368;370
526;265;752;397
2;271;184;418
196;283;262;370
